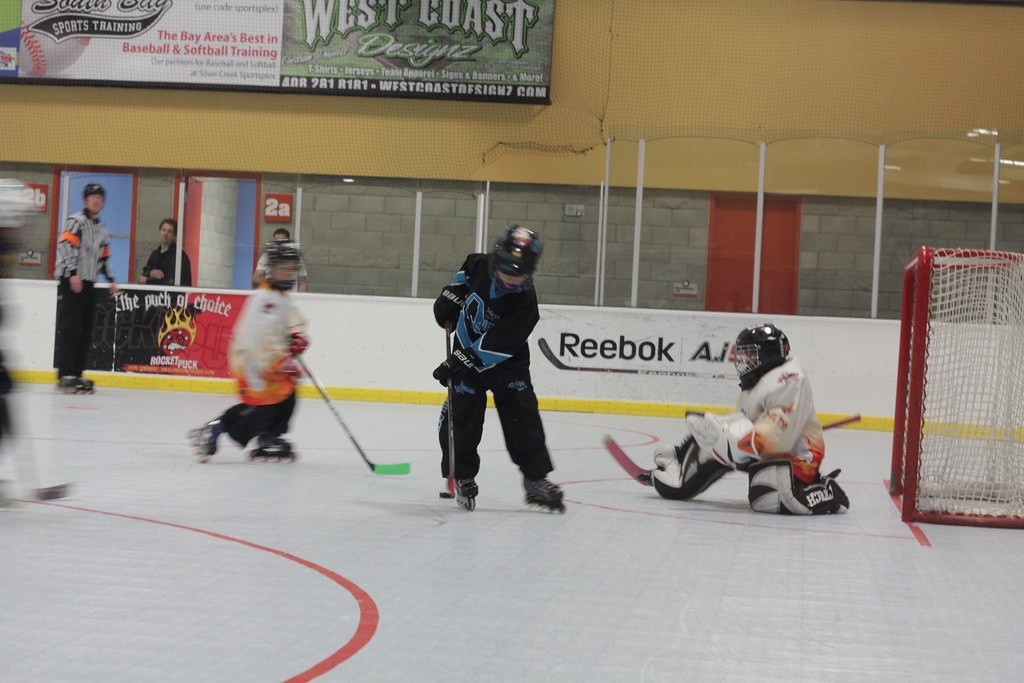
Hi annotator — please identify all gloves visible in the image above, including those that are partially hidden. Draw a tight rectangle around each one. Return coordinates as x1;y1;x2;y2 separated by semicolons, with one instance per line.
434;284;470;333
281;356;306;383
288;332;308;353
433;346;485;388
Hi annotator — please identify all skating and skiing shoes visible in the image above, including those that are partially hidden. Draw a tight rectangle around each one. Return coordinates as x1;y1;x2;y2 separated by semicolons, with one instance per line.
453;478;478;512
247;432;296;465
523;475;566;513
56;374;95;396
187;415;226;464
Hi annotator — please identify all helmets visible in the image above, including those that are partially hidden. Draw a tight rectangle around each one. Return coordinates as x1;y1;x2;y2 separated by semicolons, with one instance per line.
733;322;791;391
83;183;104;198
488;223;544;293
264;237;301;290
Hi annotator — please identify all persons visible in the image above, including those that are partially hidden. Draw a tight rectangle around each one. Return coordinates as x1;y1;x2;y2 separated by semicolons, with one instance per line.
53;184;119;394
432;224;567;514
141;219;193;286
0;178;47;507
651;323;850;515
186;228;313;463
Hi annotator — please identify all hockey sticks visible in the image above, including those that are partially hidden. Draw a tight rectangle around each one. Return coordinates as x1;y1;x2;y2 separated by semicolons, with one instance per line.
285;336;412;476
9;437;80;502
439;320;457;500
601;413;862;488
